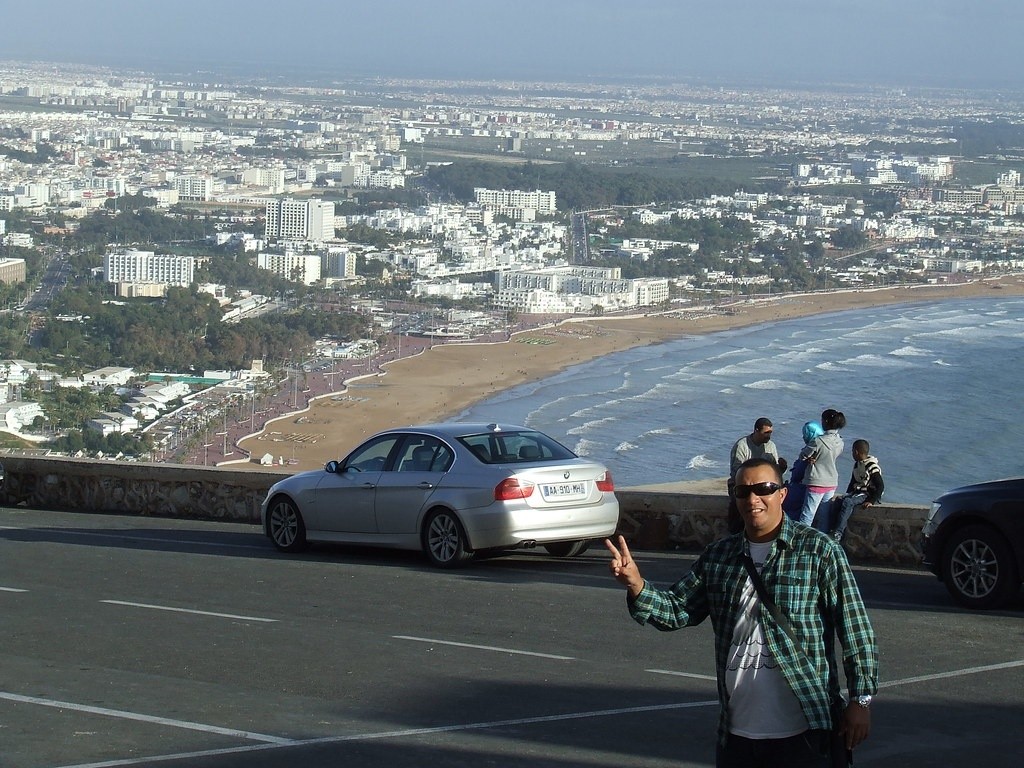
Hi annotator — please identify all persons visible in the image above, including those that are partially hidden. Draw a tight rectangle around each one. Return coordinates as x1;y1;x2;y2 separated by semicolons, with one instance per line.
606;458;880;768
727;417;788;533
784;409;846;535
831;439;886;544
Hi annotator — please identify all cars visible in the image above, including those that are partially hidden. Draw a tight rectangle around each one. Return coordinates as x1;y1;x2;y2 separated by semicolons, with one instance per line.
259;421;619;569
920;477;1024;612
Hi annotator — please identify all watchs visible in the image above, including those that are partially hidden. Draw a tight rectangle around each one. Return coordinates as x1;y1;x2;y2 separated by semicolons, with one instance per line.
851;694;872;707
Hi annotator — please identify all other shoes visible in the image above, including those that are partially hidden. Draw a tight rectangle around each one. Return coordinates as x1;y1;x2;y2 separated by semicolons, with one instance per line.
829;532;841;542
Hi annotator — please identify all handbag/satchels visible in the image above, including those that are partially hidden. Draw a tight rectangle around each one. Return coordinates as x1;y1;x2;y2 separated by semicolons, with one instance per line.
828;703;853;768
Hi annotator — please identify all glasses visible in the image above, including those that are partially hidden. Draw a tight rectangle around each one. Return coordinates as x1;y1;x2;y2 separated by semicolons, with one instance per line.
734;482;787;498
